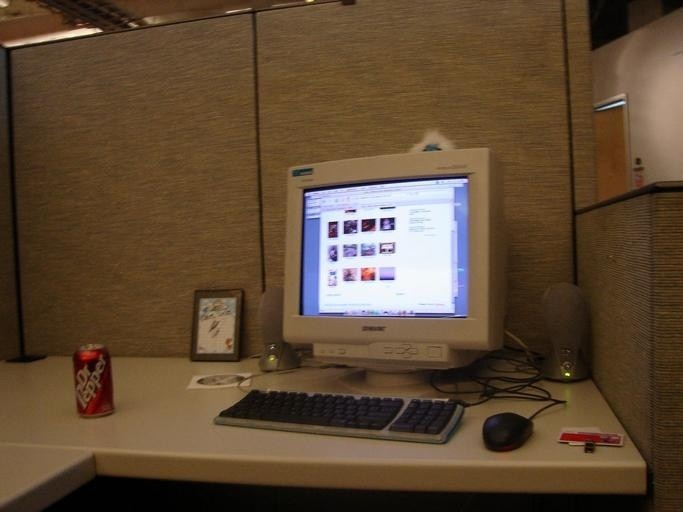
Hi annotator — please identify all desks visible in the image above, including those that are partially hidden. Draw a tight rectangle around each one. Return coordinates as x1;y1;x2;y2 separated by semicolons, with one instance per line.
1;443;98;512
0;354;649;512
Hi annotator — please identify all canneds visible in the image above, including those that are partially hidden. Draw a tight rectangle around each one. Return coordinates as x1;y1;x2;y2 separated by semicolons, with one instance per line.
72;343;115;419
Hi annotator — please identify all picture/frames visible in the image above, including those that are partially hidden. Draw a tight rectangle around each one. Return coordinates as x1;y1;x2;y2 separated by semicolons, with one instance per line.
189;288;244;363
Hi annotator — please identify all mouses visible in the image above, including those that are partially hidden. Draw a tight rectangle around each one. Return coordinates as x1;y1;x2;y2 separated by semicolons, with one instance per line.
483;413;534;451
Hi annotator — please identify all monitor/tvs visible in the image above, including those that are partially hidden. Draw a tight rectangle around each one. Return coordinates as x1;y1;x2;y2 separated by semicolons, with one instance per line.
283;147;506;396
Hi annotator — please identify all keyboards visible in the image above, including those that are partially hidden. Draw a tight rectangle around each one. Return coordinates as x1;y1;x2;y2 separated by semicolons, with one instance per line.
213;388;465;444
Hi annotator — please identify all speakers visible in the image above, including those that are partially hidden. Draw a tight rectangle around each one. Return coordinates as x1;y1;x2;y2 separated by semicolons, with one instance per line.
257;286;301;372
541;283;593;381
